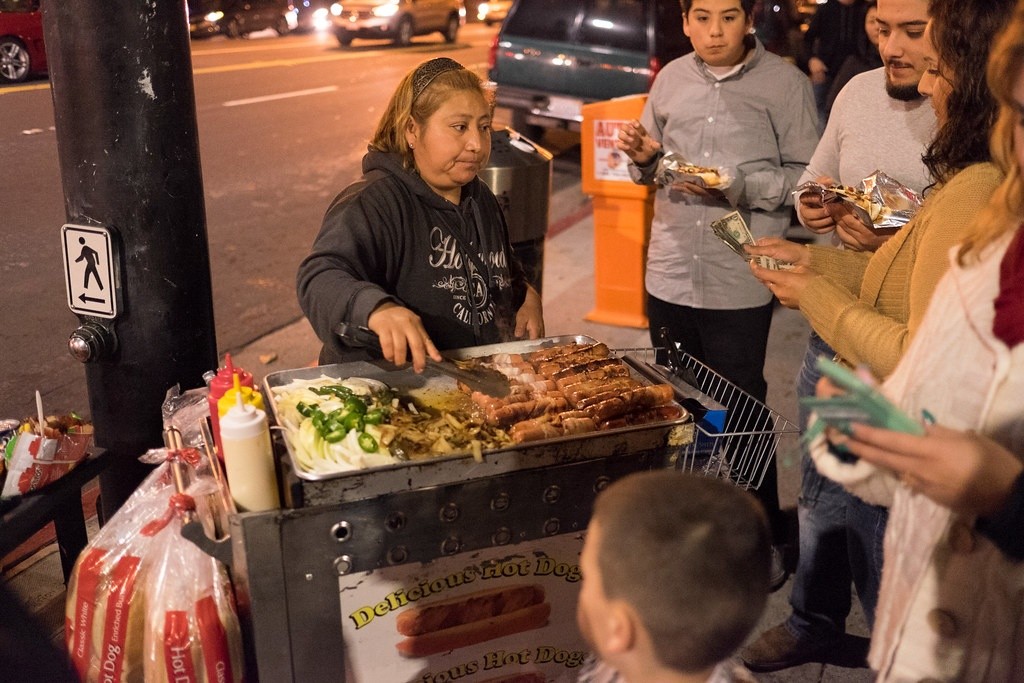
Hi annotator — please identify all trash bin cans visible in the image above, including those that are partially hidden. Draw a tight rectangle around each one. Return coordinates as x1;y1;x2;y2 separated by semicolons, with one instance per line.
478;126;554;294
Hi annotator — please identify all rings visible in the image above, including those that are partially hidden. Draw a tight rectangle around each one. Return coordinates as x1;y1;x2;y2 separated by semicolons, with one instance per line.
903;475;918;492
768;281;775;290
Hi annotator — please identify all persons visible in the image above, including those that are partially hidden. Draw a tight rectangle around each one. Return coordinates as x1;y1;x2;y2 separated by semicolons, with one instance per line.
295;57;546;376
574;466;772;683
733;0;935;672
830;3;1024;683
805;0;854;93
615;0;823;576
749;0;1014;629
824;1;878;115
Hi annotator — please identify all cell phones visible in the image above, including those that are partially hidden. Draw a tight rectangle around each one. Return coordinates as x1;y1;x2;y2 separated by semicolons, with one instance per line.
814;356;925;436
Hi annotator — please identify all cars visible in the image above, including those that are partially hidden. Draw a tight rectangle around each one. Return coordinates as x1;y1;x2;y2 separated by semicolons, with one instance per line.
189;0;301;40
0;0;50;83
480;0;514;23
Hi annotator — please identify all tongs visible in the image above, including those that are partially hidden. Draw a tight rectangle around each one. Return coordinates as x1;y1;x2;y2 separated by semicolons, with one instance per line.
338;323;511;399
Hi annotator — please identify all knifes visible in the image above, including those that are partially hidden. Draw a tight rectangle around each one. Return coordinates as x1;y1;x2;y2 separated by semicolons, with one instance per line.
660;327;700;390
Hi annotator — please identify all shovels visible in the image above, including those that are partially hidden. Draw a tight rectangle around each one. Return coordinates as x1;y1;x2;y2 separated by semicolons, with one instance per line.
659;326;700;390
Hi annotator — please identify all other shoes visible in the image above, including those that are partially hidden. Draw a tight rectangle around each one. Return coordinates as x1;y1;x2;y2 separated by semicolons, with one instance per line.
766;541;786;590
740;621;841;668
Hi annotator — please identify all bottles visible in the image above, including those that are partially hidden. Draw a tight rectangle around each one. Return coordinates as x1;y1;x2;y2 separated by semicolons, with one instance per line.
219;393;280;512
209;354;254;472
217;374;266;420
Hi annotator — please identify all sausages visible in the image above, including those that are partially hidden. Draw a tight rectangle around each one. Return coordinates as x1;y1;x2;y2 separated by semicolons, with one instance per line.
459;344;679;447
400;585;544;637
397;605;551;657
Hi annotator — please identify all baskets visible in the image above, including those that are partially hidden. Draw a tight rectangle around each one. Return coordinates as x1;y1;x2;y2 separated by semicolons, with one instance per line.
608;343;801;493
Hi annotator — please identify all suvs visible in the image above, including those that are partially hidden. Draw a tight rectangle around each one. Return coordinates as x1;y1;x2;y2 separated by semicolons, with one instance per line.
485;1;684;139
327;0;468;47
